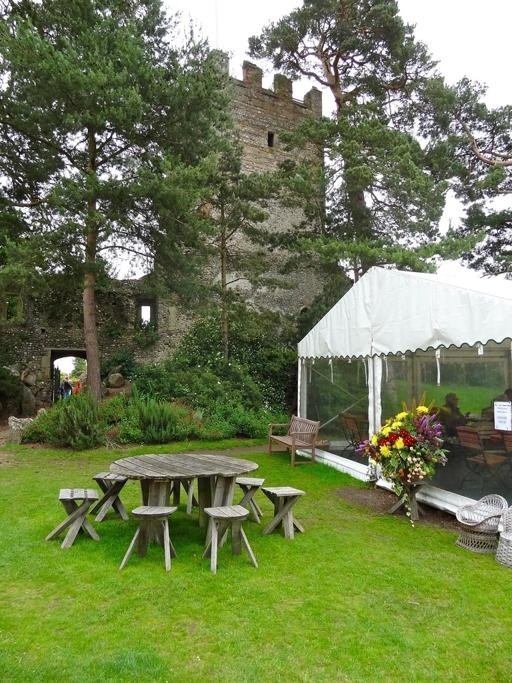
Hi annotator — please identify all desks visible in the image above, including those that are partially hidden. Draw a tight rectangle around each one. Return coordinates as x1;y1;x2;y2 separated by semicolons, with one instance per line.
107;452;260;559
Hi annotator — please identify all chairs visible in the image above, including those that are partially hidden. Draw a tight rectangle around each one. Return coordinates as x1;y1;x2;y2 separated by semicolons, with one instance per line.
452;490;508;553
423;389;512;499
493;503;512;569
336;410;366;462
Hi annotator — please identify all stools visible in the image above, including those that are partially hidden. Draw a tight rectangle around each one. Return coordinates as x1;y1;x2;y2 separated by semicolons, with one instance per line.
118;505;181;576
94;468;131;524
169;482;201;511
237;472;269;527
200;501;258;577
258;482;309;541
43;485;103;550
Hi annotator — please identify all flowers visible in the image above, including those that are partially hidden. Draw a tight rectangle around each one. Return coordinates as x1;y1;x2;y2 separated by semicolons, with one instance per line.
353;395;456;532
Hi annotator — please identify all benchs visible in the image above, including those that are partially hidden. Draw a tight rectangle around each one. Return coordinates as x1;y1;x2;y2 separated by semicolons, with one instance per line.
265;412;321;469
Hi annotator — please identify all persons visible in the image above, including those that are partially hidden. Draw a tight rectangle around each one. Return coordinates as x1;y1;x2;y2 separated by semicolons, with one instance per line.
442;392;469;429
61;375;81;398
494;388;511;403
